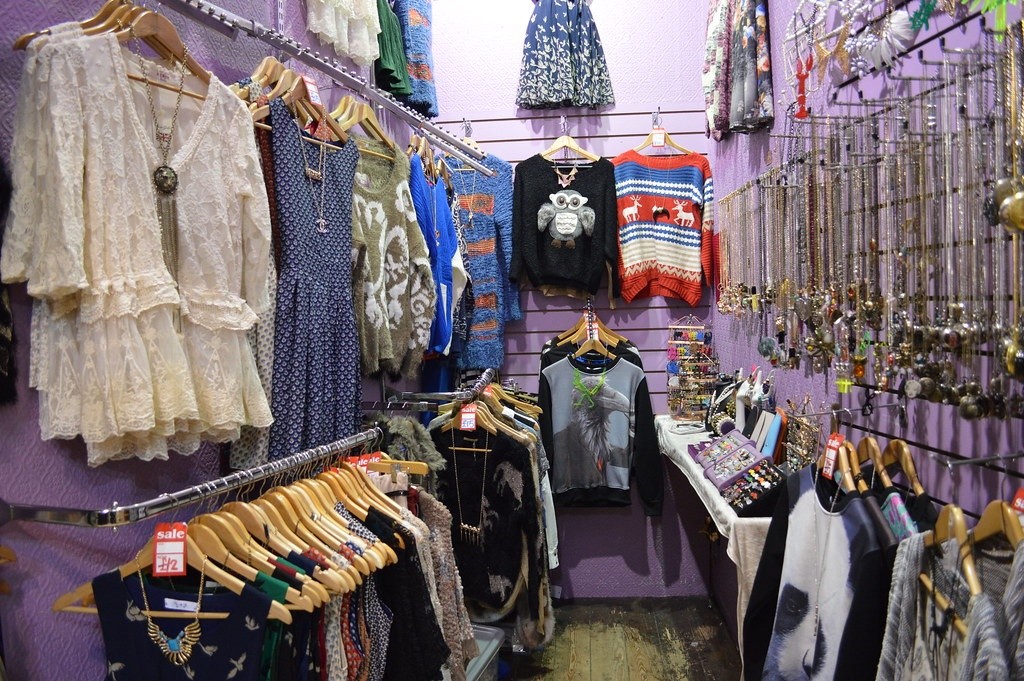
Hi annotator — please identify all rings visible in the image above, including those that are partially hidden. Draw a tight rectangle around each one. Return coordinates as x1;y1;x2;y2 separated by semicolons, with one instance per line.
702;433;783;509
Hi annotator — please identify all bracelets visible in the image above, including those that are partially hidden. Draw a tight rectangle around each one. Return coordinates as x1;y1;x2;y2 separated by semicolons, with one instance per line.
709;411;737;436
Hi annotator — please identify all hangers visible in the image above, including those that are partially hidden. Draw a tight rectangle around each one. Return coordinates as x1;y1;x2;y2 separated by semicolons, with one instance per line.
542;121;600;169
410;122;453;190
968;454;1024;552
633;114;692;155
437;370;543;446
816;406;925;497
919;459;981;640
227;32;349;150
557;303;629;362
328;84;395;162
52;427;428;624
444;121;487;171
13;0;210;101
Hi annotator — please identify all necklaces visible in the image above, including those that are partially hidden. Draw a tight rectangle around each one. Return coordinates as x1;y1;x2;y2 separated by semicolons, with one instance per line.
716;0;1024;421
549;143;581;188
572;342;609;409
454;155;476;229
286;88;329;235
869;463;877;490
905;474;918;506
167;546;233;595
430;150;441;247
449;414;489;547
707;383;738;424
128;18;190;333
813;450;851;637
646;136;674;221
931;518;975;678
134;545;207;668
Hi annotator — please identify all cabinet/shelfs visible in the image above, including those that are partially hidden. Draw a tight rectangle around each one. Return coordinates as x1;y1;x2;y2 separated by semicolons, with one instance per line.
668;315;720;419
